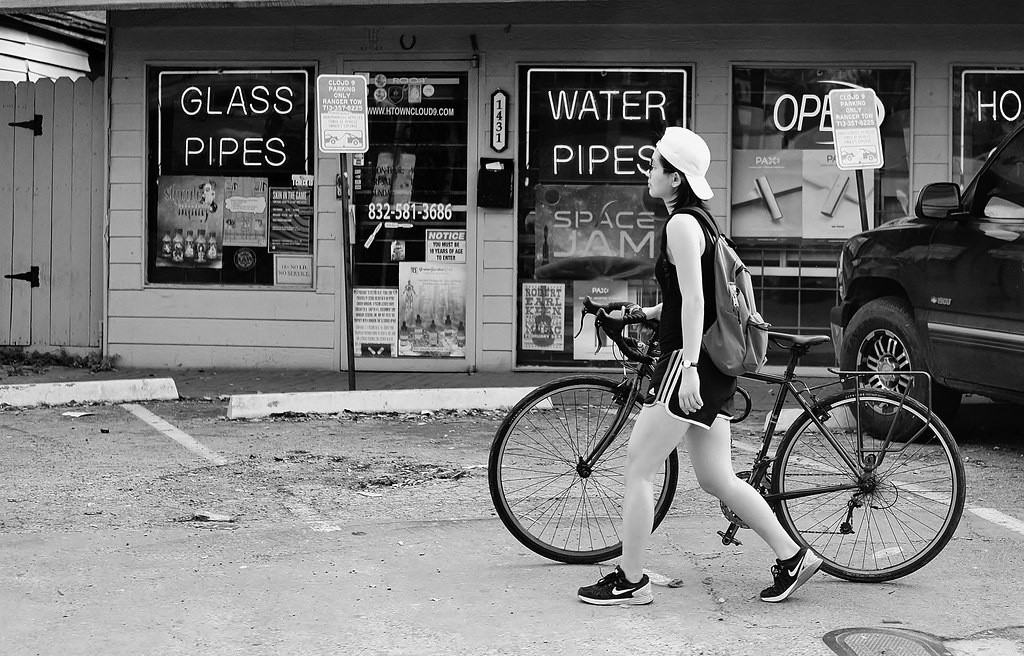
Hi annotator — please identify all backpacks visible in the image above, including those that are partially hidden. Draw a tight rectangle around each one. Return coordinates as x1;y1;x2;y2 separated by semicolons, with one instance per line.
671;205;772;376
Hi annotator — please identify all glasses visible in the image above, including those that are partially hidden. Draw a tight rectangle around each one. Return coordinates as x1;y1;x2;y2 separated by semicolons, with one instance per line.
647;159;669;173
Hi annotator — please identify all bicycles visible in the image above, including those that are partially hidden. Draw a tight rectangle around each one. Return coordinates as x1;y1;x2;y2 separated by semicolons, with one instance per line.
486;293;968;583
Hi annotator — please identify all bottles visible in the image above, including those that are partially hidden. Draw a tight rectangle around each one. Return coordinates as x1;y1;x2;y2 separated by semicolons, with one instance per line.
172;228;184;262
162;230;173;257
208;230;217;260
184;230;194;258
194;229;208;263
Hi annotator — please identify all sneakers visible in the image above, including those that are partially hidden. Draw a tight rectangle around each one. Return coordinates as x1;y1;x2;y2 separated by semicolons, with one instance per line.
577;564;654;605
758;547;823;603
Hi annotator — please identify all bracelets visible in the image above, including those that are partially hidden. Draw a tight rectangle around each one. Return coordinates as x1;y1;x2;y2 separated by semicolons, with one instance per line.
681;361;699;370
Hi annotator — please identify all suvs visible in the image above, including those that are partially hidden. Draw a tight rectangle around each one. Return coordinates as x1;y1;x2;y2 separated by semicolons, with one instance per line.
829;117;1024;445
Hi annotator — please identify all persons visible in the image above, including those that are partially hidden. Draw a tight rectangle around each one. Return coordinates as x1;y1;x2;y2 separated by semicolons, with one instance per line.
575;126;826;607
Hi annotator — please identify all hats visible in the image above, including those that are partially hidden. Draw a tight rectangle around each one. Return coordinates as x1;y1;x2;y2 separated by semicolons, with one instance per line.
655;126;714;201
